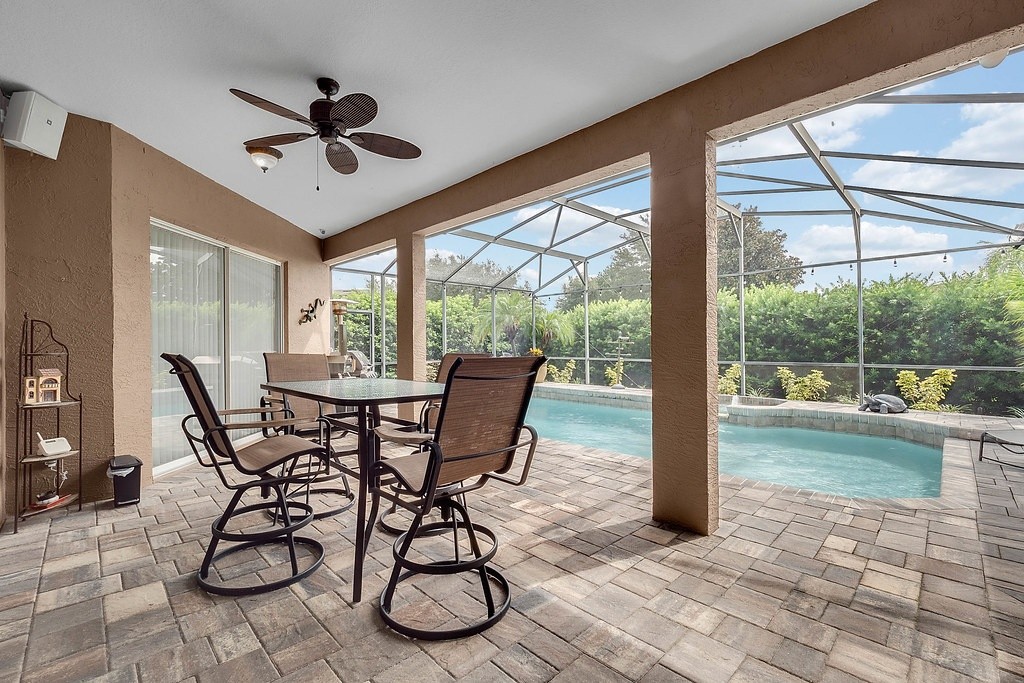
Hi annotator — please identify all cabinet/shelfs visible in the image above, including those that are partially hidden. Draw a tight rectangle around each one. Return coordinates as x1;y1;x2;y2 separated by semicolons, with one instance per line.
15;313;83;534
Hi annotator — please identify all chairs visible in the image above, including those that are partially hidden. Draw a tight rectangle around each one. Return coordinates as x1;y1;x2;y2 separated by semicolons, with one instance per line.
158;352;325;595
378;354;550;641
379;352;503;537
258;352;357;520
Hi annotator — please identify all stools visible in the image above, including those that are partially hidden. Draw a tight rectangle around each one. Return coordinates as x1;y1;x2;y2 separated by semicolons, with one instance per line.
980;428;1024;471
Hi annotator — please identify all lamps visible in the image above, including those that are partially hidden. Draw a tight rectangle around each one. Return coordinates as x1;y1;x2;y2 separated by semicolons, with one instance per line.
250;146;283;173
318;125;339;144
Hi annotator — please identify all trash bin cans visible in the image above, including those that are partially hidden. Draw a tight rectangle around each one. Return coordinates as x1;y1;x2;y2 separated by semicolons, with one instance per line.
109;454;144;507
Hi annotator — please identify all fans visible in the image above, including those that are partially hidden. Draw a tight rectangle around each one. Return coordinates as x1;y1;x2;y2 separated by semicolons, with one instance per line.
229;76;421;174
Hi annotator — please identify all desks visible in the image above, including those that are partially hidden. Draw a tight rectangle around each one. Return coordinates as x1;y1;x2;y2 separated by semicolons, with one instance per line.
261;380;447;602
